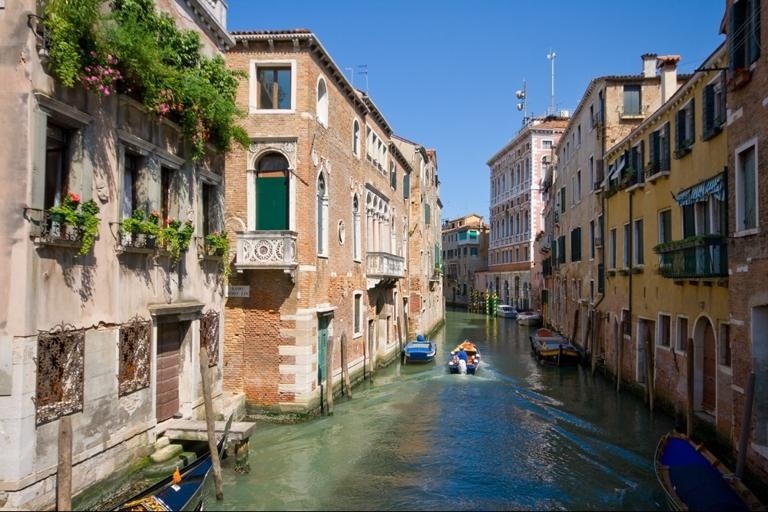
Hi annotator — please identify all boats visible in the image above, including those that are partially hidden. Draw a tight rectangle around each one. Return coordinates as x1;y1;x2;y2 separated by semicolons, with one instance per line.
654;424;766;512
403;332;437;363
496;303;518;319
515;310;542;326
528;326;580;370
446;336;482;374
111;408;234;512
